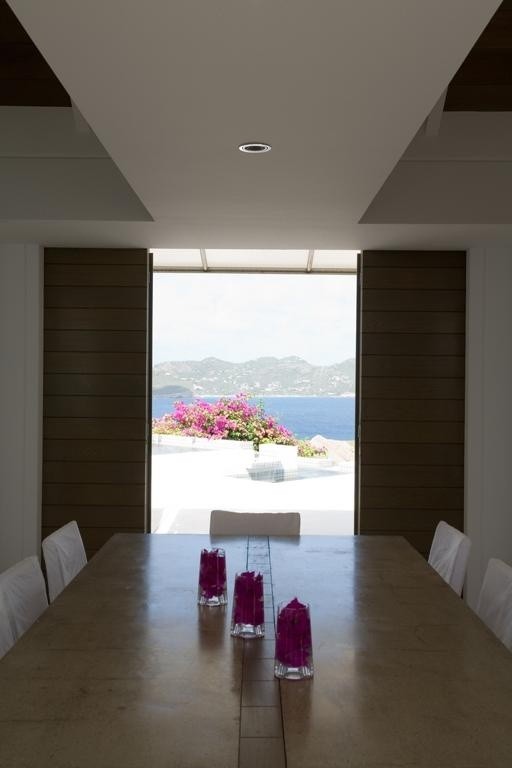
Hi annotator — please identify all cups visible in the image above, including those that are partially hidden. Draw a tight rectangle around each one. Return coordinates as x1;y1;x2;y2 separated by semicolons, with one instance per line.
196;546;228;608
272;601;316;681
229;571;267;640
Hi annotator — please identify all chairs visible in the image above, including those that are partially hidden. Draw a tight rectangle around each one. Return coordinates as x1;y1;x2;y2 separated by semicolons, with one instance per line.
1;556;50;664
473;556;510;656
42;519;88;605
425;521;471;597
209;510;300;538
